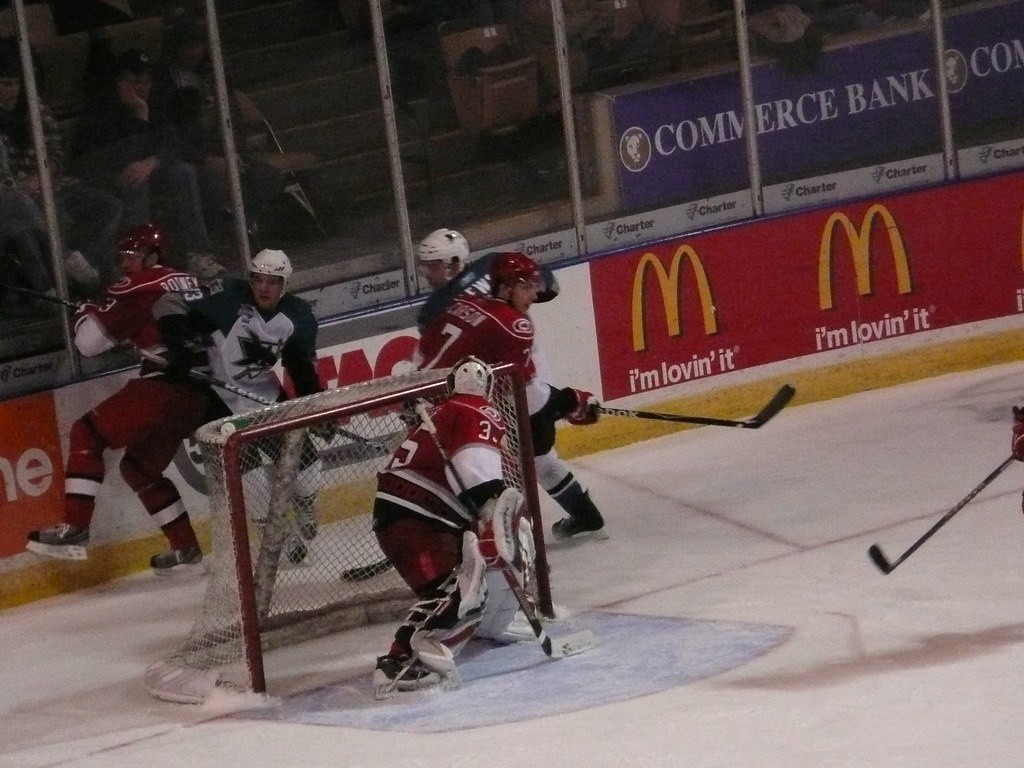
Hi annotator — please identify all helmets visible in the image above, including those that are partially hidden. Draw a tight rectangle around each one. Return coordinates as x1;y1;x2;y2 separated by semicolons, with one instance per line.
246;248;293;299
418;228;469;280
488;252;542;297
110;219;171;264
445;355;495;403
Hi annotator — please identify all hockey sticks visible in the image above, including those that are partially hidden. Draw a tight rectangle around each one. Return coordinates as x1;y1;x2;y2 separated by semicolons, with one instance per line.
134;345;390;454
417;402;597;659
341;557;394;582
867;454;1016;575
0;281;81;309
596;384;795;429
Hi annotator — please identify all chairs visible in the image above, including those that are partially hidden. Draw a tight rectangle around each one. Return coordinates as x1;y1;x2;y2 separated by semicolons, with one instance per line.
0;2;1024;319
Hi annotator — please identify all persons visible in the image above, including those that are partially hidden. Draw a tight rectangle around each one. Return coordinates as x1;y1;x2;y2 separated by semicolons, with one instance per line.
24;222;337;577
1;0;294;310
369;356;514;692
406;231;610;543
365;0;685;165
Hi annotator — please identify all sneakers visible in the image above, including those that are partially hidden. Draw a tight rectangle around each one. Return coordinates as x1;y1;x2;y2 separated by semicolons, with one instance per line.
25;521;90;561
552;488;609;543
373;652;441;700
149;545;205;577
250;517;312;568
65;251;103;284
292;492;320;542
29;289;75;317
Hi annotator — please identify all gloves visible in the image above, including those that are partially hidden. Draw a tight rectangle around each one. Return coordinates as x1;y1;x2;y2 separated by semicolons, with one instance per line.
479;520;509;571
399;400;420;426
70;297;99;334
308;420;338;444
561;387;600;426
162;342;192;383
